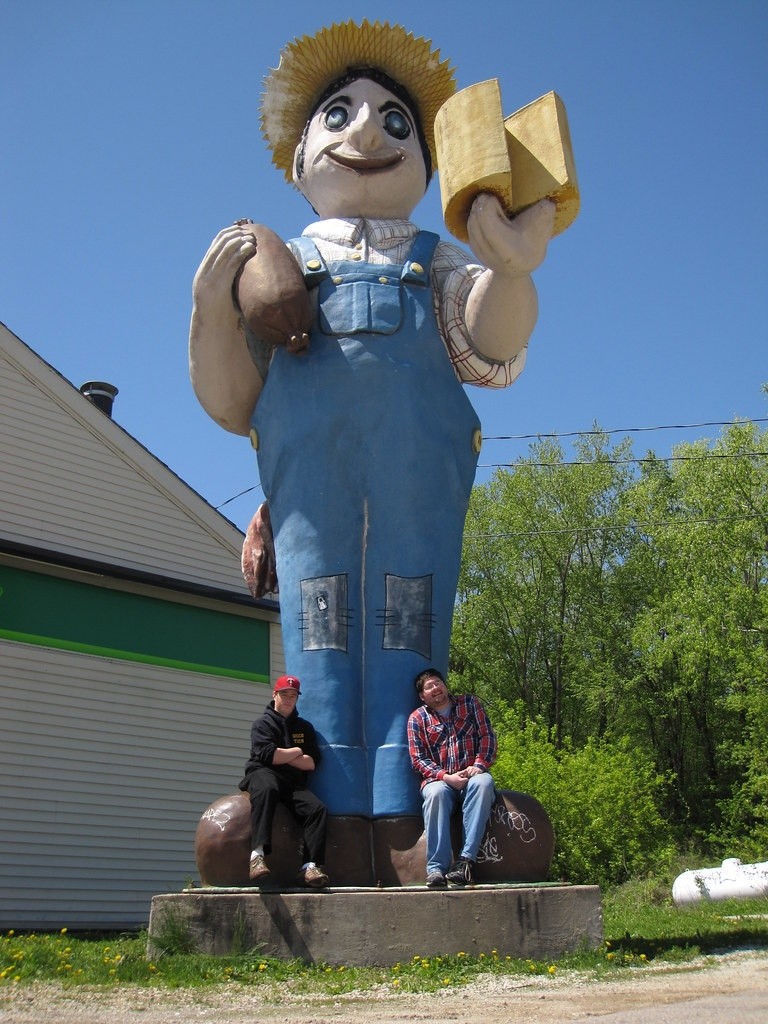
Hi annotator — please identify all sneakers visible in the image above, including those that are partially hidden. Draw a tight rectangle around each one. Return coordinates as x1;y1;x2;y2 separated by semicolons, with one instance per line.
293;866;329;889
427;872;446;886
446;860;473;886
249;855;272;883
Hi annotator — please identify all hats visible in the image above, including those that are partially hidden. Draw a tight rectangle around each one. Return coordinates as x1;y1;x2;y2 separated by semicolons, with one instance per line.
274;677;302;696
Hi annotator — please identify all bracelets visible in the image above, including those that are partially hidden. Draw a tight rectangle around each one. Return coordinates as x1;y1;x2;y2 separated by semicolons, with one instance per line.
473;764;486;772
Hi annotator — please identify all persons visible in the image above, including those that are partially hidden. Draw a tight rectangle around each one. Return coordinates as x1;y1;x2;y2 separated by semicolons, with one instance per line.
239;677;329;887
189;20;556;818
408;669;497;885
